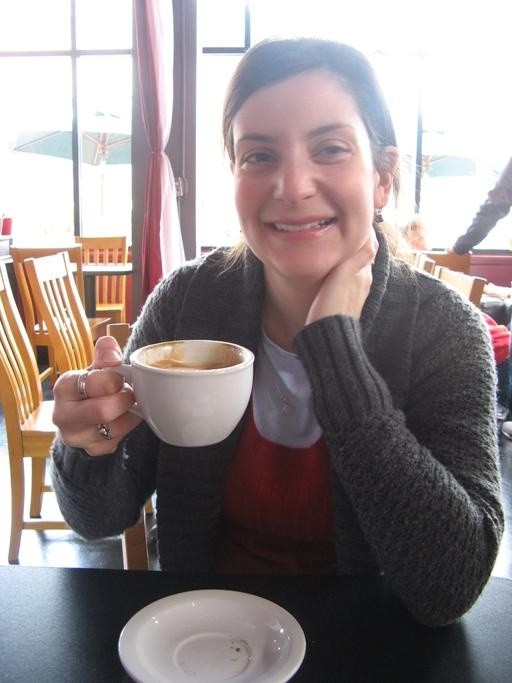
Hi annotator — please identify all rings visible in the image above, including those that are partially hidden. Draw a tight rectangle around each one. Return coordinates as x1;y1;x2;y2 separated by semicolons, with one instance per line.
77;371;90;401
97;421;113;443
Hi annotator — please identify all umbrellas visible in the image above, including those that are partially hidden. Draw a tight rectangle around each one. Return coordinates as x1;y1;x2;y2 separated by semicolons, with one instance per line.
14;108;138;167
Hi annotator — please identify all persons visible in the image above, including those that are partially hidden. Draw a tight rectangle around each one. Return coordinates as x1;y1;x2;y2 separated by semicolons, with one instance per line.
47;34;505;632
441;157;512;255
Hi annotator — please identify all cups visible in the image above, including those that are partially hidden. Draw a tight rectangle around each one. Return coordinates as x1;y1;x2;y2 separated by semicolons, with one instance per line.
100;339;256;448
1;218;12;236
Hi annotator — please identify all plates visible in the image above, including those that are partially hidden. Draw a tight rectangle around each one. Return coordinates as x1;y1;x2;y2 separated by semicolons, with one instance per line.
117;588;308;683
